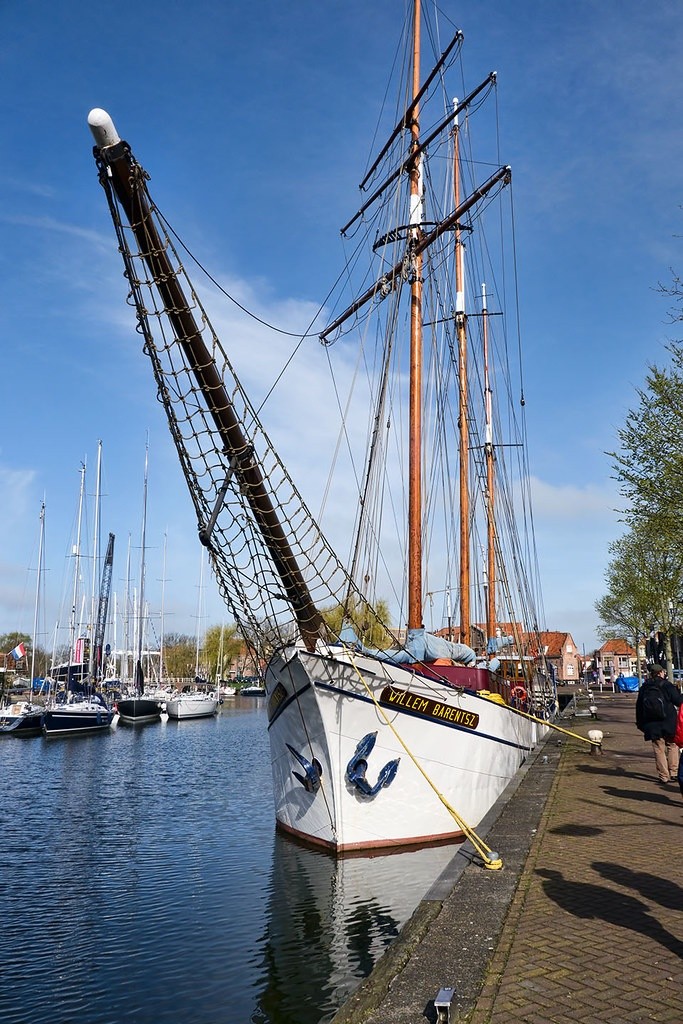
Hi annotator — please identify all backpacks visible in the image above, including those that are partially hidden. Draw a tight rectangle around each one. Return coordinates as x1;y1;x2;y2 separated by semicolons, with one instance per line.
641;678;667;722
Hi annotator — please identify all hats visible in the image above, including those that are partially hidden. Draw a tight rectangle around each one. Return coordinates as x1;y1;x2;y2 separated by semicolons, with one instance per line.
650;664;665;672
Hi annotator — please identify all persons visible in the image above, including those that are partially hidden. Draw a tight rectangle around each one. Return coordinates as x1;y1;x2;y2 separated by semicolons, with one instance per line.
673;695;683;803
634;665;683;784
194;673;265;684
591;669;625;684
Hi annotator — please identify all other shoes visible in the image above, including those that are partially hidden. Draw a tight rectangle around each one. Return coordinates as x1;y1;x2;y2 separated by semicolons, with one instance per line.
669;777;678;782
657;779;667;783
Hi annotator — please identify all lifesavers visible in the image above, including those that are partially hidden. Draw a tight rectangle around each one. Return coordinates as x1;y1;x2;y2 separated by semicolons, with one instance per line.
511;685;528;700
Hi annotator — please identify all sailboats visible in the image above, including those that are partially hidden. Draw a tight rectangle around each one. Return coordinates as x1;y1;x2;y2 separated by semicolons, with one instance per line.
85;2;559;854
0;426;265;735
